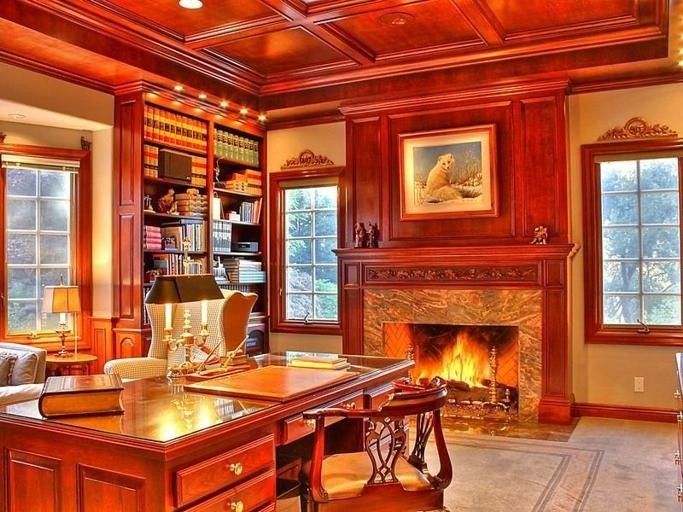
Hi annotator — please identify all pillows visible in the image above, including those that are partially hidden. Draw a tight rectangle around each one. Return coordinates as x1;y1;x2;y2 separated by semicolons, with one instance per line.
0;349;19;385
10;352;41;386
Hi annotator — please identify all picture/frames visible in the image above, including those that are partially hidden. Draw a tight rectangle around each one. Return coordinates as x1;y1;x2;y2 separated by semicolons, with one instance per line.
397;124;499;221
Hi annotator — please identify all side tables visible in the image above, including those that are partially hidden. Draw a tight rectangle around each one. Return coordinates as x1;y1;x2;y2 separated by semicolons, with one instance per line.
46;353;97;376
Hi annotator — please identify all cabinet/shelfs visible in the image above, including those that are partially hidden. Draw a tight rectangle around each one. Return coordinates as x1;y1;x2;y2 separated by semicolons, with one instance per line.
111;79;268;359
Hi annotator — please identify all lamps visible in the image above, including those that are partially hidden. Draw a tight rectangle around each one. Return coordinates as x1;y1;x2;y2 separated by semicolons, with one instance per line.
42;286;80;358
144;275;224;377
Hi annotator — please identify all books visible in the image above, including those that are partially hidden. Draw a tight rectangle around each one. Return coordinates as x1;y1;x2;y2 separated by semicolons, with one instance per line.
292;355;348;370
38;372;125;418
142;103;268;324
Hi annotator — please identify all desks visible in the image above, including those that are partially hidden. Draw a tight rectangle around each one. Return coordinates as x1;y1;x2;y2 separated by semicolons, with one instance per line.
0;353;416;512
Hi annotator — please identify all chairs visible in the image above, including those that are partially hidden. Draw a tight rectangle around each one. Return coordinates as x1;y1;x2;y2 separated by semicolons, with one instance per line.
103;289;258;382
299;376;452;512
0;342;47;406
